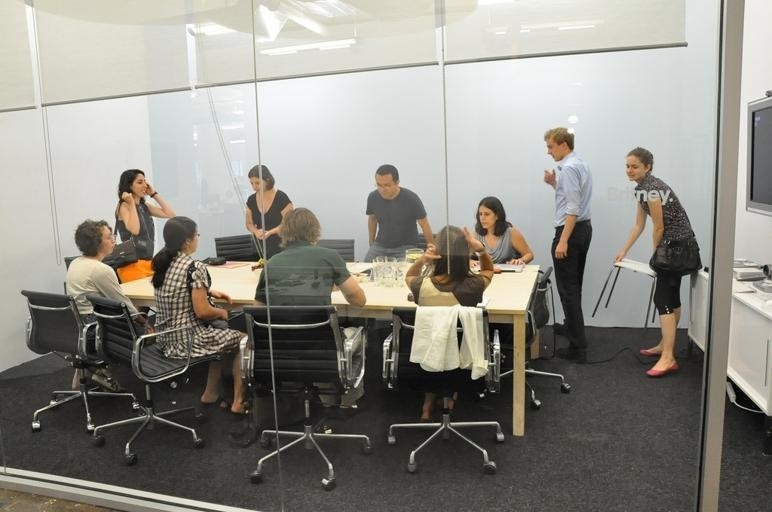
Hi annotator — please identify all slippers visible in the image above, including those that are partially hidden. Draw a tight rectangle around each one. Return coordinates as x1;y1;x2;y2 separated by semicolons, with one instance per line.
228;404;253;417
200;394;232;414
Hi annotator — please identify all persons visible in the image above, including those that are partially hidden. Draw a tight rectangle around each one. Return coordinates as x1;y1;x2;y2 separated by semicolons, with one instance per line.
468;196;535;269
610;147;703;379
404;223;494;421
243;163;297;263
362;163;437;263
252;207;370;416
542;127;595;366
115;168;176;317
65;217;156;392
150;216;252;416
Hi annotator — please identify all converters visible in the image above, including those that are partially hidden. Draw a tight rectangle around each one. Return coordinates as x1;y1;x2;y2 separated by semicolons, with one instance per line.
726;382;736;402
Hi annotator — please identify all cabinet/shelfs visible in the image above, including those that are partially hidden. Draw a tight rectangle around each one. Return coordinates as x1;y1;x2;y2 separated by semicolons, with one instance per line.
683;265;772;420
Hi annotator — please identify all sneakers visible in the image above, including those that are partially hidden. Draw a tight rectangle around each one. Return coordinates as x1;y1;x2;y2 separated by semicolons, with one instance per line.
92;367;127;392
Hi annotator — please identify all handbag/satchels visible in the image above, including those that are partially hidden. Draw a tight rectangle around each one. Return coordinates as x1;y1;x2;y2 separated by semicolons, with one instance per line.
102;239;138;269
649;238;704;273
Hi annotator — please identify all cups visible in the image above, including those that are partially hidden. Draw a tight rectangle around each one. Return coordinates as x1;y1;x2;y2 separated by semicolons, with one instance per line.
404;247;426;271
373;256;397;289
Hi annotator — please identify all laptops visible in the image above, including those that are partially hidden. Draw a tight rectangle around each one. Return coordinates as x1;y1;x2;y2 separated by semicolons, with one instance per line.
472;264;523;273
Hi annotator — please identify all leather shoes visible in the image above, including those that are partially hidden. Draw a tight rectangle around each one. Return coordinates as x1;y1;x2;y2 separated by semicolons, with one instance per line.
645;362;680;377
555;345;588;364
640;347;679;359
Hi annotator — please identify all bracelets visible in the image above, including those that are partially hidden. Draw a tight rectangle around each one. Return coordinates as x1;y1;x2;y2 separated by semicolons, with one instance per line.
148;191;159;198
475;240;487;254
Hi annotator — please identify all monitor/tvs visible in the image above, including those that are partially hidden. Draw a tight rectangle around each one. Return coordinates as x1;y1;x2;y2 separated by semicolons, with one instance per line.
743;96;772;218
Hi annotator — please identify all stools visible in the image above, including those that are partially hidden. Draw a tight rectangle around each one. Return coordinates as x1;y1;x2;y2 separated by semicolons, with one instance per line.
590;258;660;330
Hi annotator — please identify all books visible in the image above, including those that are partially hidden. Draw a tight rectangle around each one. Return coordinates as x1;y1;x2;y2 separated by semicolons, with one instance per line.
216;260;250;269
472;261;525;273
